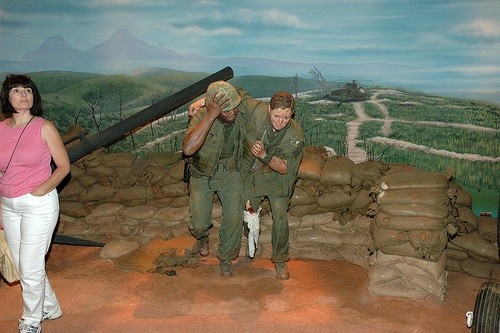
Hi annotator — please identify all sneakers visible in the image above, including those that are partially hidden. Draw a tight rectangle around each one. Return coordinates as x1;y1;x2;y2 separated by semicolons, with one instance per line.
220;259;233;277
40;305;62;322
274;261;289;280
18;319;42;333
198;237;209;256
245;239;256;256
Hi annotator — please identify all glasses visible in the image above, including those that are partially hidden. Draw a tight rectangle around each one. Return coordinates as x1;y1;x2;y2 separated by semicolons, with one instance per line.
6;74;31;80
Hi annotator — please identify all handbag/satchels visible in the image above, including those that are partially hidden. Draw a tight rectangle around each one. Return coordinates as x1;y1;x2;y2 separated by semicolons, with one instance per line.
0;229;19;284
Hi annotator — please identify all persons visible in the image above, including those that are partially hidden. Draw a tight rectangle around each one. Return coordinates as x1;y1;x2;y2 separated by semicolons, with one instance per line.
0;73;70;333
187;86;304;279
182;81;258;277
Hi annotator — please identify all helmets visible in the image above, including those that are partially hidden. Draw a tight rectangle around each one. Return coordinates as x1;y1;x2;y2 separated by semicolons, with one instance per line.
206;80;242;113
